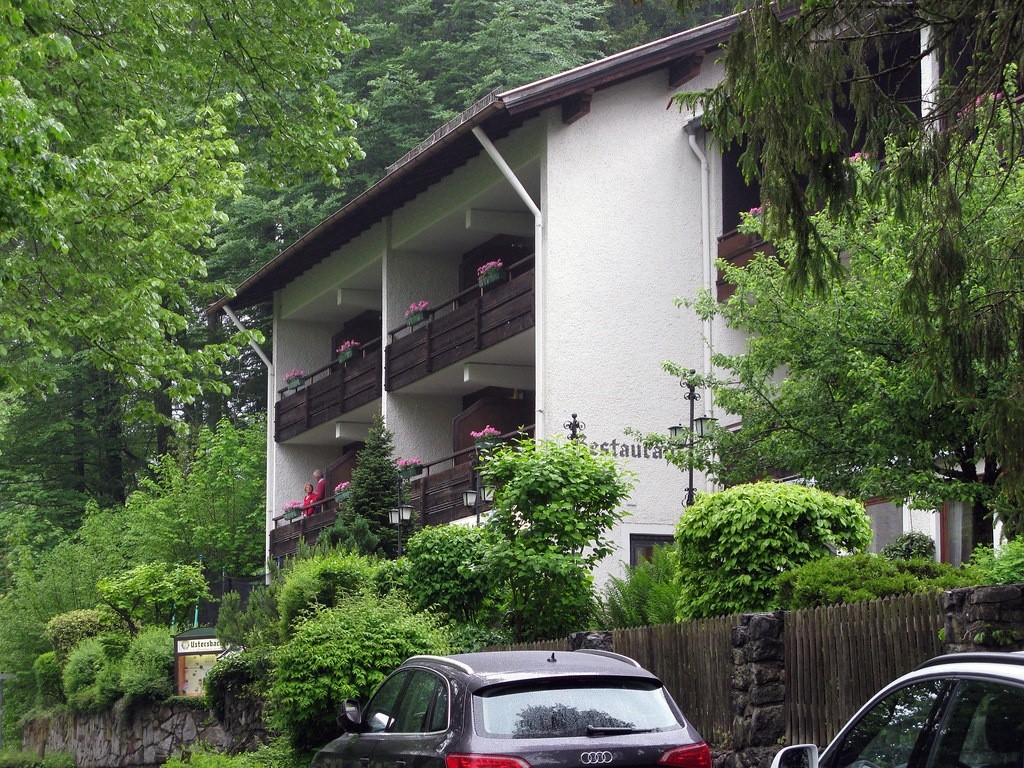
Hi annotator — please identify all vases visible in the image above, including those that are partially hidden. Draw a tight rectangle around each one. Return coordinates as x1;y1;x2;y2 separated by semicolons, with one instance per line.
287;378;305;390
334;489;352;503
474;437;500;450
399;465;423;478
283;510;301;521
406;310;428;326
478;271;502;287
338;347;358;364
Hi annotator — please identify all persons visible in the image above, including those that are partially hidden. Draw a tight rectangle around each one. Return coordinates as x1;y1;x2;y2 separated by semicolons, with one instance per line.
309;470;325;516
302;483;317;517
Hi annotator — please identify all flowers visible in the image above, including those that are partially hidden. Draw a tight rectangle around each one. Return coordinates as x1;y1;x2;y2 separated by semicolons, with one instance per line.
477;259;503;280
397;457;421;469
333;481;351;495
282;501;304;512
472;425;501;443
850;152;871;164
336;339;360;354
283;369;305;384
404;300;429;319
740;206;764;221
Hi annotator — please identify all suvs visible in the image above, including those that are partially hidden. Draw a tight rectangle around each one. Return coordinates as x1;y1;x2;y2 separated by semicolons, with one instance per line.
308;648;713;768
770;647;1024;768
